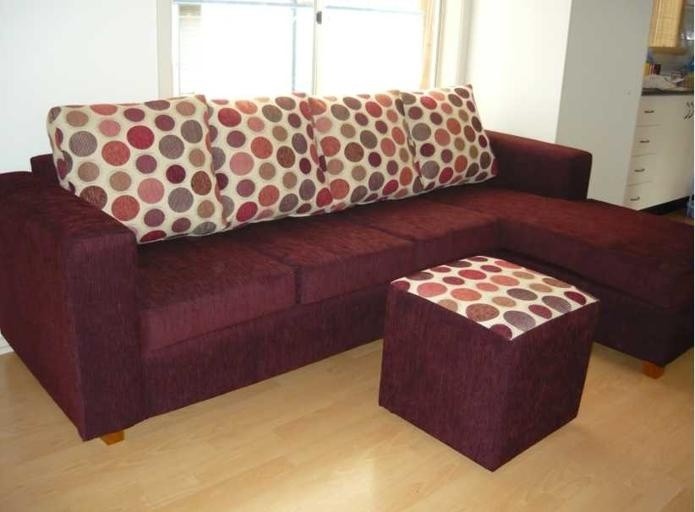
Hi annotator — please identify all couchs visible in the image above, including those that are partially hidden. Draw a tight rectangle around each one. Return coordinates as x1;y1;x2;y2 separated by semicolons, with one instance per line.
0;130;694;442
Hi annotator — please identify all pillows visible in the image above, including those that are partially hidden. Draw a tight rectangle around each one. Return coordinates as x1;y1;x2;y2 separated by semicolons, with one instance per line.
206;92;336;232
309;91;425;211
47;95;228;245
400;81;498;194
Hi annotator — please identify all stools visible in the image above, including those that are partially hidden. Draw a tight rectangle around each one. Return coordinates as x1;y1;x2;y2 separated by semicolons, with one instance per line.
379;255;600;471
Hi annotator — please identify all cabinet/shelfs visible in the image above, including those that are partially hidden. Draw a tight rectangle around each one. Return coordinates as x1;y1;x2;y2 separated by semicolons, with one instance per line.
624;86;694;211
